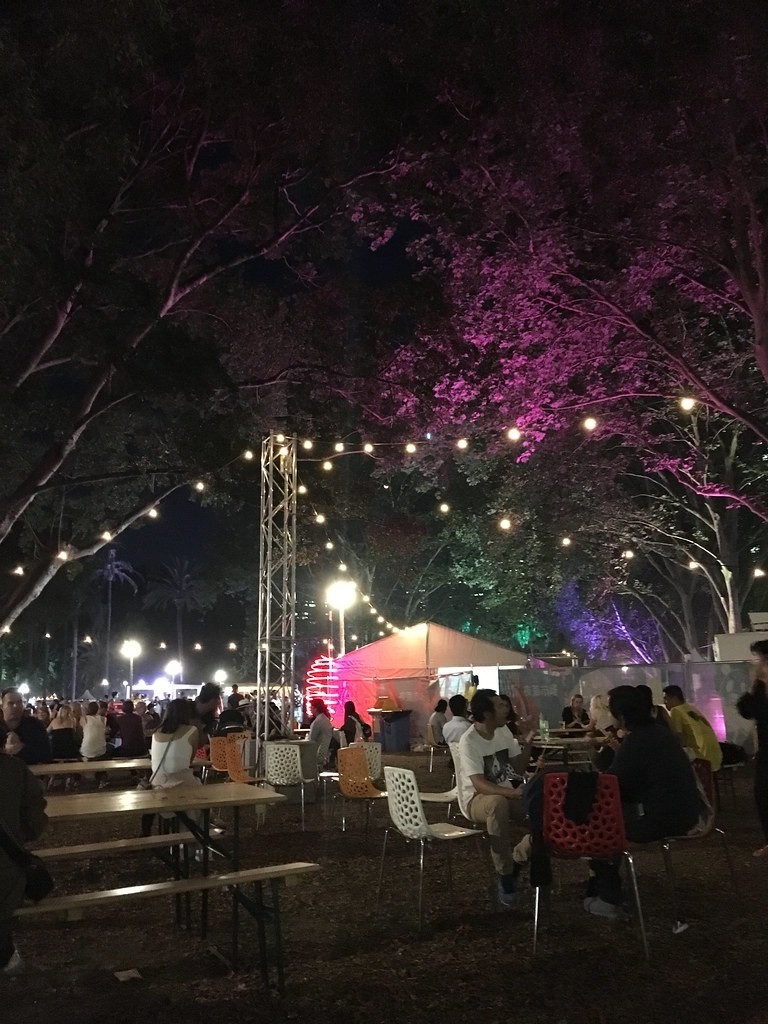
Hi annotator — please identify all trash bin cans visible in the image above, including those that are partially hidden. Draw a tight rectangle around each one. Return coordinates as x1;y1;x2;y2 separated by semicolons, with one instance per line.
380;708;412;754
366;696;399;742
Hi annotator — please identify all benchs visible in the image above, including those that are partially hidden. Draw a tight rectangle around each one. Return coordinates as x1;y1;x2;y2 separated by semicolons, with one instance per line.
31;827;222;931
12;862;320;991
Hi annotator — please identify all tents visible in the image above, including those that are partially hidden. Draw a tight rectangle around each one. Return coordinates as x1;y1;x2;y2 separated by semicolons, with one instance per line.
327;620;559;745
77;689;102;707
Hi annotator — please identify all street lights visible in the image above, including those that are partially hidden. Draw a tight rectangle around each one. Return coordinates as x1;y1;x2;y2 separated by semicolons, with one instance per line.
126;643;141;694
329;583;353;658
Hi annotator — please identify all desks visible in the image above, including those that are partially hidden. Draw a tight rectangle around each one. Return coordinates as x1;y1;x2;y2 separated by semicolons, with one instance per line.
44;782;286;932
539;727;593;736
27;759;152;777
530;738;613;773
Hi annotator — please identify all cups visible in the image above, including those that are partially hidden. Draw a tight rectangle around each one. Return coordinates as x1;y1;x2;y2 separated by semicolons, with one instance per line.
558;721;565;729
540;720;549;741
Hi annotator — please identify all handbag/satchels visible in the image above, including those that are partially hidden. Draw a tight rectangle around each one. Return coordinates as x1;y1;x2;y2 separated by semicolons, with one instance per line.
348;715;363;743
137;776;152;790
25;850;54;902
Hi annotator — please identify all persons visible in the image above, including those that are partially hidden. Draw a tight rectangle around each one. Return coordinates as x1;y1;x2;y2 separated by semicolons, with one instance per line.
329;701;367;769
561;694;590;738
428;694;538;778
0;724;48;972
633;685;723;773
459;689;545;907
25;685;333;791
736;639;768;857
141;683;227;839
0;688;51;766
582;685;716;920
581;695;617;772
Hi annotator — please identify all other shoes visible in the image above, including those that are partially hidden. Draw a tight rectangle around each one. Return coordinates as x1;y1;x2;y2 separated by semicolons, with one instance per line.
753;844;768;857
583;896;617;919
0;950;24;977
171;845;188;860
495;858;522;897
194;850;216;863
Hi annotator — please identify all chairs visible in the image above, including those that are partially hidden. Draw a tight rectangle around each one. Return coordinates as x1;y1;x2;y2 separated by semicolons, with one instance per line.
209;730;267;829
418;724;745;961
256;744;314;831
374;766;494;934
337;748;388;832
318;745;328;767
320;743;382;823
